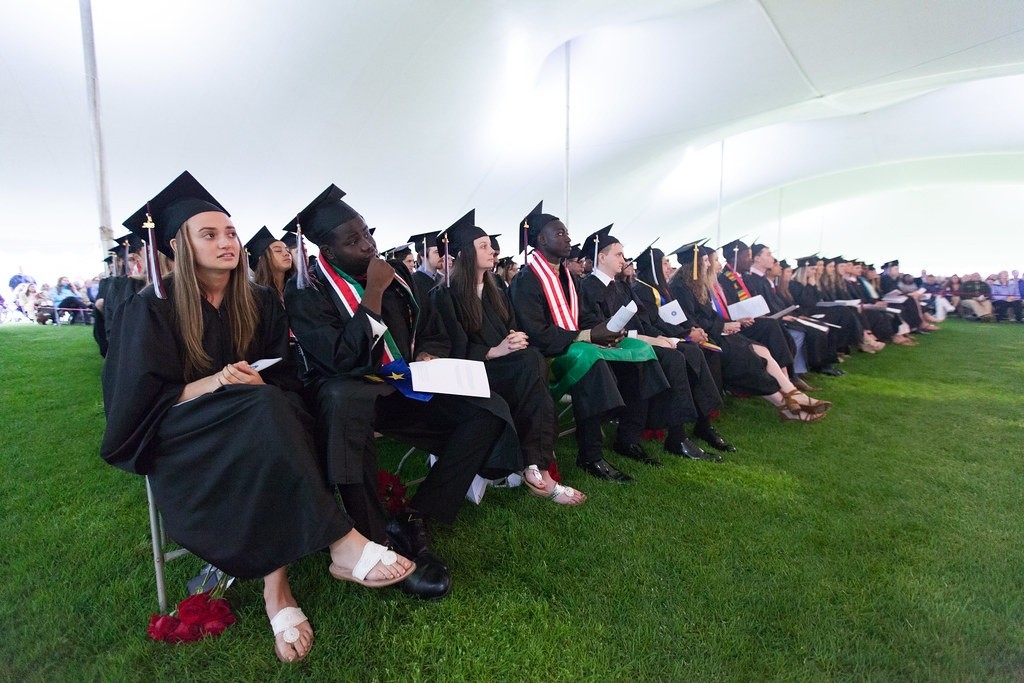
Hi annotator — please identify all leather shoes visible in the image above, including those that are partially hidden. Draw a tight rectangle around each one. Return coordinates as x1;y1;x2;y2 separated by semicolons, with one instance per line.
614;443;663;466
382;539;453;597
665;437;723;463
794;378;822;391
575;457;635;481
694;425;738;452
818;368;841;376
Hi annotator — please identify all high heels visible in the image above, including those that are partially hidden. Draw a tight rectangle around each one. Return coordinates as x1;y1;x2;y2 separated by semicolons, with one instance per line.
785;390;832;415
779;398;827;423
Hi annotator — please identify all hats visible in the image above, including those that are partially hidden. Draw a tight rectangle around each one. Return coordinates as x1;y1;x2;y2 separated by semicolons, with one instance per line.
122;169;231;298
283;184;364;288
577;223;619;274
519;201;559;265
749;237;767;259
105;169;908;279
716;235;752;275
437;208;488;288
623;237;665;285
795;256;818;268
666;238;709;280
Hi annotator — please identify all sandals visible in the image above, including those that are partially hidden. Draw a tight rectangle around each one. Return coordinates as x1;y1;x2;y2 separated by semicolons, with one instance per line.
521;465;549;495
546;482;587;505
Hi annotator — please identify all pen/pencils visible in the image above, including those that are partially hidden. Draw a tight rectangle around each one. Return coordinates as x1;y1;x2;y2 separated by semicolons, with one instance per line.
250;365;258;370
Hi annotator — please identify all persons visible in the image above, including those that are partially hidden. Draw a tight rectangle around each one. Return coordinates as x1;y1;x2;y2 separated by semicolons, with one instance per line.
0;263;100;326
91;168;1024;666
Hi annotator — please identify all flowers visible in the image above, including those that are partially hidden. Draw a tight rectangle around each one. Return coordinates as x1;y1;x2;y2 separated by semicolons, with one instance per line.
377;464;416;525
546;460;564;485
638;425;667;442
141;558;239;646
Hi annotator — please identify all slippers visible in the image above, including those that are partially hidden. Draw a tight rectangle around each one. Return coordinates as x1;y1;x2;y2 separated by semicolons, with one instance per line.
269;608;316;659
330;542;417;588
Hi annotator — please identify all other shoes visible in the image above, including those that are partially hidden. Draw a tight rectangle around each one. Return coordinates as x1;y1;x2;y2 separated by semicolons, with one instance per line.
821;323;940;378
